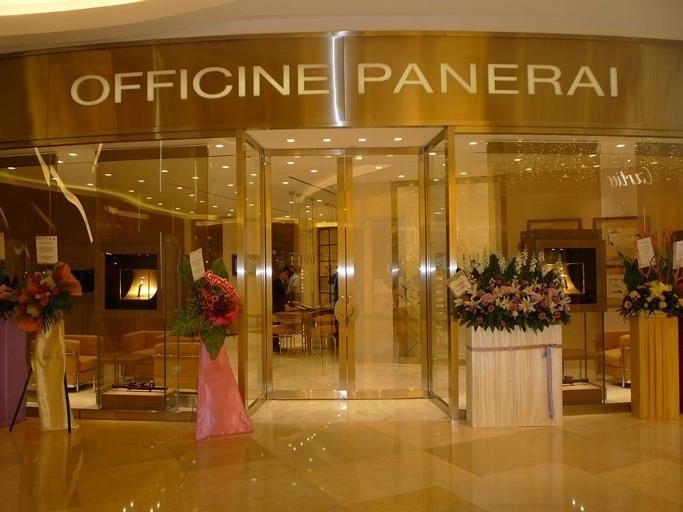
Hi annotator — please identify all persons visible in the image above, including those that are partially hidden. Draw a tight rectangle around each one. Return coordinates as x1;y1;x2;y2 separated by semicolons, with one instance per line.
285;268;301;308
328;267;338;339
272;269;291;352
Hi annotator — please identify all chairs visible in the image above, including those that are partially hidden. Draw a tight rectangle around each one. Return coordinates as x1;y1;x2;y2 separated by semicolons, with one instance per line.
115;326;173;387
60;331;104;392
266;298;335;358
595;325;634;386
148;336;202;404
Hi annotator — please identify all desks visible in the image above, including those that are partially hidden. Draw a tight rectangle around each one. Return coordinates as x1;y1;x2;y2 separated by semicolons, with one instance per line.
94;351;152;395
555;347;605;404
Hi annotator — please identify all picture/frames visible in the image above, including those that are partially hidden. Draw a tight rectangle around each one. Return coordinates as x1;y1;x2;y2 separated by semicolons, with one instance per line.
589;215;650;270
533;236;609;319
102;250;158;313
524;218;583;231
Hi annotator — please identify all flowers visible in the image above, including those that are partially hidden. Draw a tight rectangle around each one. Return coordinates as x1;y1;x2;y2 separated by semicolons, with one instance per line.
170;263;242;353
617;281;683;319
451;250;576;334
0;265;76;336
